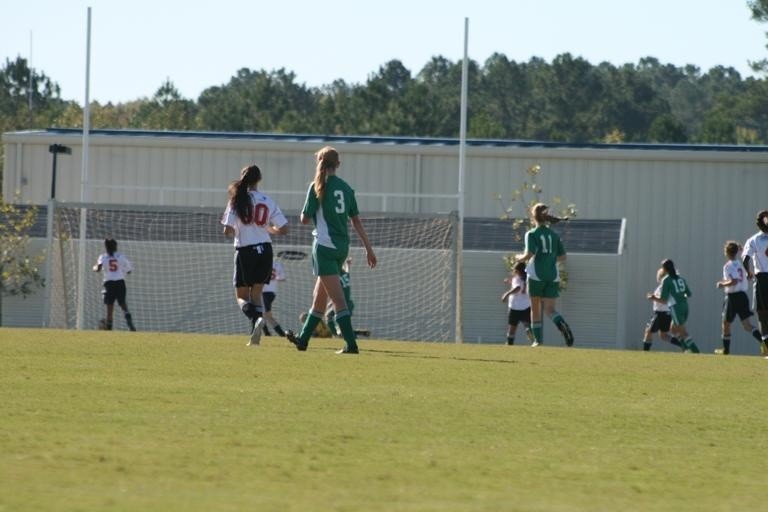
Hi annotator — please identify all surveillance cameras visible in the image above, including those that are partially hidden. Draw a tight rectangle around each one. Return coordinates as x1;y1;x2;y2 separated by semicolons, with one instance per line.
49;144;72;155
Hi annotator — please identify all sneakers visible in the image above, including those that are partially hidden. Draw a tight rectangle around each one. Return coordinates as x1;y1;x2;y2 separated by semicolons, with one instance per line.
246;315;265;347
559;321;573;346
284;330;308;350
760;341;767;356
715;347;730;354
334;346;359;354
530;341;543;347
127;321;136;331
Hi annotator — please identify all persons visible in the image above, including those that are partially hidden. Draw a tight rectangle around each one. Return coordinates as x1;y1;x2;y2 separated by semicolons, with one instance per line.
514;203;575;347
714;241;768;357
646;257;699;353
92;238;138;333
284;146;378;355
741;210;768;357
325;257;358;339
220;164;289;346
262;257;286;337
642;267;683;354
502;261;533;346
299;311;333;339
